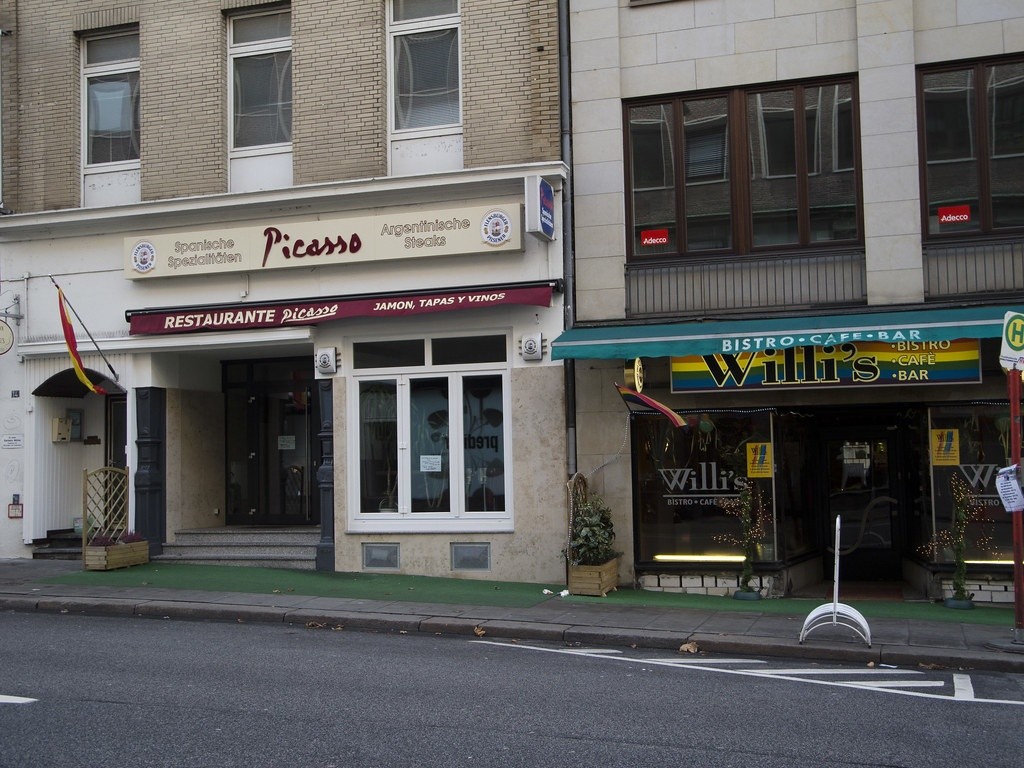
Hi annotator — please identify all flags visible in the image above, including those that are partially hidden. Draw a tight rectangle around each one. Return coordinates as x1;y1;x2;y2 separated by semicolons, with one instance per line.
617;385;688;426
58;289;107;395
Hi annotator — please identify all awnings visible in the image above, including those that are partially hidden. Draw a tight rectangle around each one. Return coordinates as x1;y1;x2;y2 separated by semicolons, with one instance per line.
128;285;553;335
550;302;1024;361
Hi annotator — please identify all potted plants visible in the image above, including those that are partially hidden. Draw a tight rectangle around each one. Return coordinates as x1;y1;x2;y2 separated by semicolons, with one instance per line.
943;488;975;609
733;492;761;600
567;492;623;596
85;531;150;569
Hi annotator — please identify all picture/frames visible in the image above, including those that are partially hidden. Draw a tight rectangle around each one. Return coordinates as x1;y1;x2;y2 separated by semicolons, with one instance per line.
65;408;85;441
